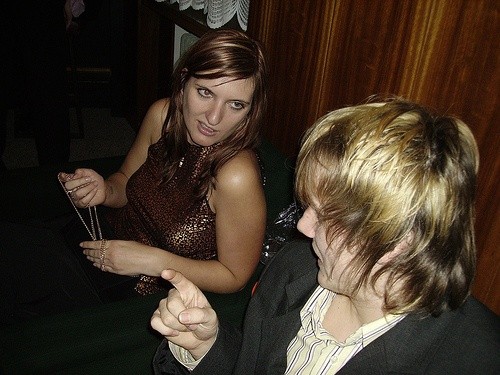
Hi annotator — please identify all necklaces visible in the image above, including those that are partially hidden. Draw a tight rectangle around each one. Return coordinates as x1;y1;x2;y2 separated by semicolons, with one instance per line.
178;154;186;167
94;204;107;272
57;171;98;243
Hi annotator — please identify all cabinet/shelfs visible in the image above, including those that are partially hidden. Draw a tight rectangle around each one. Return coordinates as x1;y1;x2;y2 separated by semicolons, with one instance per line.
124;0;500;318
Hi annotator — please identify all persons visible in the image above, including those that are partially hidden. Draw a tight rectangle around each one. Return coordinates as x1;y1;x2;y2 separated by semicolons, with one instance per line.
150;91;500;375
0;29;273;325
